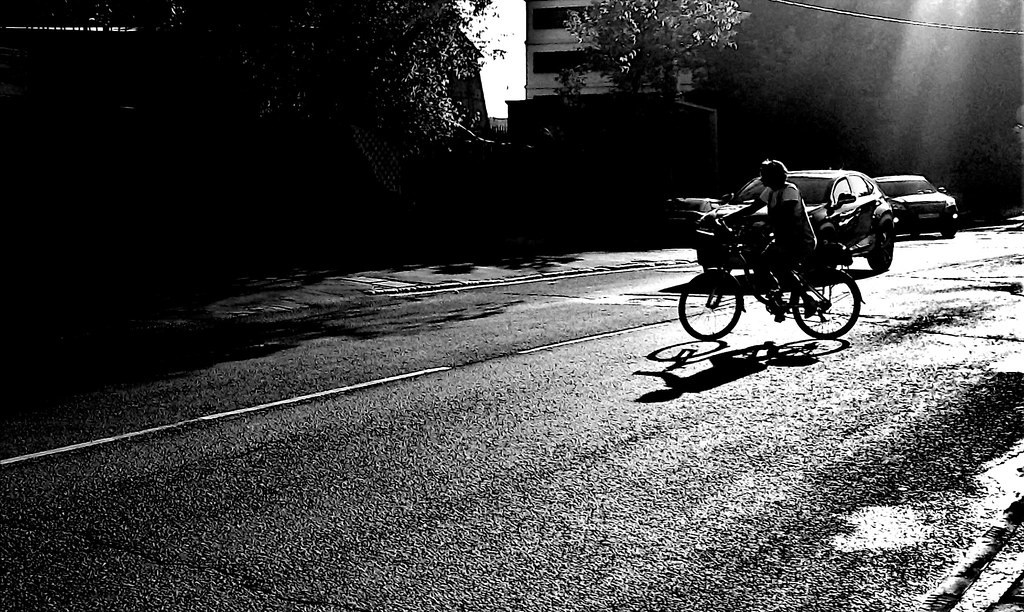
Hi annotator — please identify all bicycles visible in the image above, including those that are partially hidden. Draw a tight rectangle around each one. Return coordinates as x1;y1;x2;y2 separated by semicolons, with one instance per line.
679;214;868;340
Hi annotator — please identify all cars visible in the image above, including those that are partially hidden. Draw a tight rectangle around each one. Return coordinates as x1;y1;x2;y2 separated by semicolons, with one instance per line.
873;174;967;239
696;169;896;275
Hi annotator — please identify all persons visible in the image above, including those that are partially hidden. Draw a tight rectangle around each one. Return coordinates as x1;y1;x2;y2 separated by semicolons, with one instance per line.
723;158;817;323
